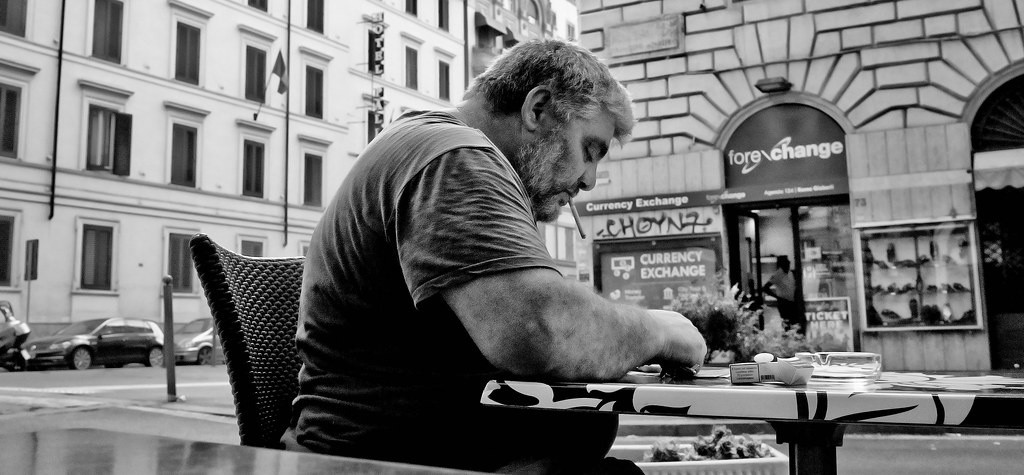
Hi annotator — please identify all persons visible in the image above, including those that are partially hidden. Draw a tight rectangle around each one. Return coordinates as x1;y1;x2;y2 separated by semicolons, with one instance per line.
762;254;795;324
291;39;708;475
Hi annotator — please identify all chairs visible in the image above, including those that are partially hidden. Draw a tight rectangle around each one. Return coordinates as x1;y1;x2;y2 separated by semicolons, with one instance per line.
188;233;309;451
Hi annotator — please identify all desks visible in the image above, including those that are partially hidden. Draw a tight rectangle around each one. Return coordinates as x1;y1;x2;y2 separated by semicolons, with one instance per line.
0;427;513;475
480;367;1024;475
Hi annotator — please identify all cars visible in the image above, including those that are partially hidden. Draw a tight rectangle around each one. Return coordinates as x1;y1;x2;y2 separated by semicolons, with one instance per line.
23;316;165;367
174;317;227;365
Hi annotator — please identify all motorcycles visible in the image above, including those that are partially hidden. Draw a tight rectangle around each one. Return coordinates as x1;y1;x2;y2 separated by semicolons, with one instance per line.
1;300;32;372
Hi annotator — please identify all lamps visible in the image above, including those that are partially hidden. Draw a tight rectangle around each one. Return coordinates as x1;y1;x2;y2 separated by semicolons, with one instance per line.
754;76;793;97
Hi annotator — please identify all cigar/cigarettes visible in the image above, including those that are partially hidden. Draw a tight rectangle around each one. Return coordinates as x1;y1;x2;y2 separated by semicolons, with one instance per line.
568;195;587;241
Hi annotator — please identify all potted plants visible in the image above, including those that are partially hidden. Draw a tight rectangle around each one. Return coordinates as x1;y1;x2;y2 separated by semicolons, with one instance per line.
605;425;789;475
